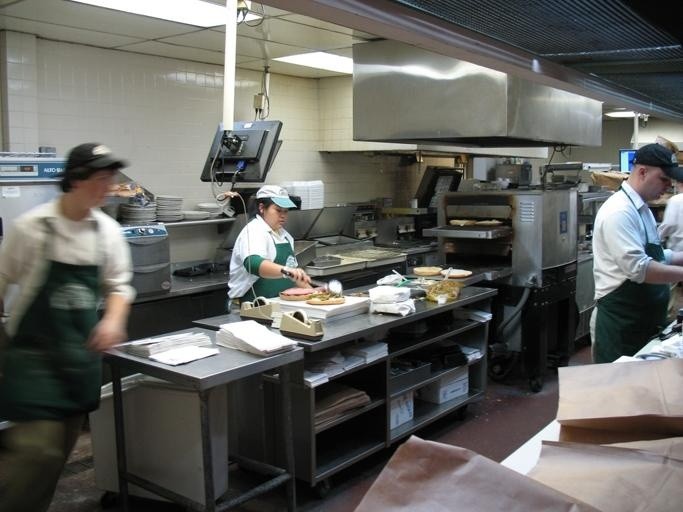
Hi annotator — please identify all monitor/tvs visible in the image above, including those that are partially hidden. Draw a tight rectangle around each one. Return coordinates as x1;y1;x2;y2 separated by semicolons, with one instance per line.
200;121;283;182
620;149;638;173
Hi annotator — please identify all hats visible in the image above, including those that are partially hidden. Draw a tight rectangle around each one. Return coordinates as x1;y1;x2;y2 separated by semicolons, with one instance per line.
256;184;296;211
64;143;128;177
629;144;683;181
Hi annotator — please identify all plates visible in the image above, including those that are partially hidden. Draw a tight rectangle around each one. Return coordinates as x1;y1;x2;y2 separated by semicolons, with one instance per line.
413;266;472;278
448;220;503;226
307;297;345;304
280;180;325;210
102;195;223;224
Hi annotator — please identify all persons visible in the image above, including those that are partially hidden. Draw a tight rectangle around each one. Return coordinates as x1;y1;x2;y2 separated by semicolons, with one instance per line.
657;167;681;316
587;140;682;361
226;186;313;312
0;143;139;512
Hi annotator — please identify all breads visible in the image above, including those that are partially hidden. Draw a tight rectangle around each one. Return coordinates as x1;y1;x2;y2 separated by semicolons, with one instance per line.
450;219;475;225
476;219;503;226
440;269;473;278
413;266;441;276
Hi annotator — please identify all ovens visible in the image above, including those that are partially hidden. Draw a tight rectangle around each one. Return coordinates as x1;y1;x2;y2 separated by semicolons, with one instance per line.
410;189;577;288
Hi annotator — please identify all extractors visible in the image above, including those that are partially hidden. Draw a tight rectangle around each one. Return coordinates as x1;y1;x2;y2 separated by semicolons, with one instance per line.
353;40;601;147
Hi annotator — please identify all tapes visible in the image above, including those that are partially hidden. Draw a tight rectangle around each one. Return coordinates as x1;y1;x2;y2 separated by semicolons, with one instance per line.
291;309;308;324
252;296;270;308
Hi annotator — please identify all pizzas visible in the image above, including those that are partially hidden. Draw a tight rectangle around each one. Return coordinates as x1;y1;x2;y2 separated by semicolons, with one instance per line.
306;293;344;304
279;287;314;300
